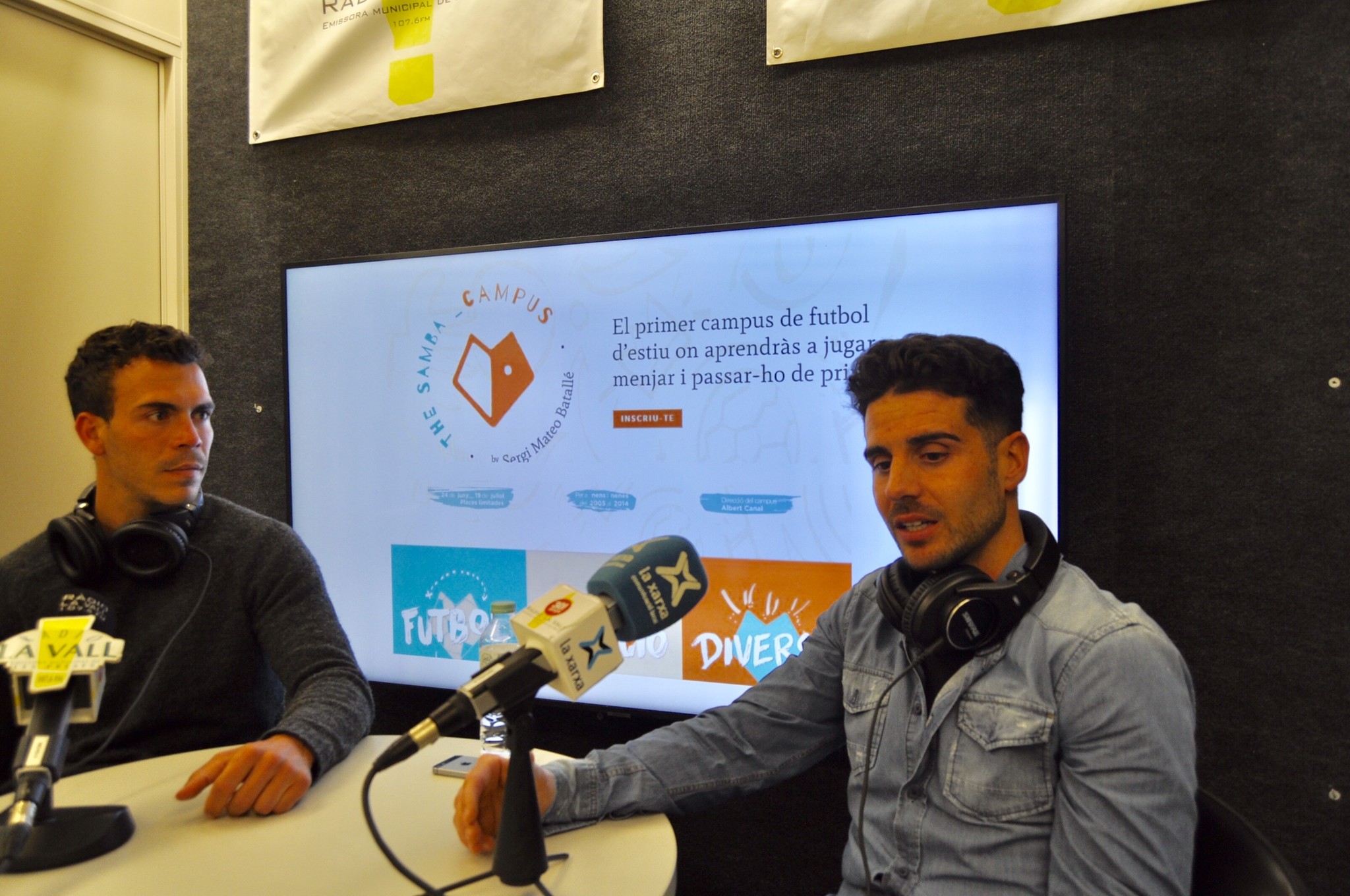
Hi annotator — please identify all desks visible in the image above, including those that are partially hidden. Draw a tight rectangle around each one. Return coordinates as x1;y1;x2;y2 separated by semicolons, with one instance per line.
0;735;678;896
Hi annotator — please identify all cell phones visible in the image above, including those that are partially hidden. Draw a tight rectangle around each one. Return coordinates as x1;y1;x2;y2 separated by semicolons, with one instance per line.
432;752;478;780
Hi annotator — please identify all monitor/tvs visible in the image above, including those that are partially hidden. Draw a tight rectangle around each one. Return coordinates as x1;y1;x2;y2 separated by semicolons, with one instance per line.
281;191;1066;722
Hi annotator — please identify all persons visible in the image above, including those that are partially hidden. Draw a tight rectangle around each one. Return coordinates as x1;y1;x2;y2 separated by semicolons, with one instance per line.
454;332;1199;896
0;321;376;818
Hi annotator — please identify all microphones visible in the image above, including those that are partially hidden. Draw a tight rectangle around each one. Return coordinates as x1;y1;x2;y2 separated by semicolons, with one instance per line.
0;588;126;836
372;534;709;772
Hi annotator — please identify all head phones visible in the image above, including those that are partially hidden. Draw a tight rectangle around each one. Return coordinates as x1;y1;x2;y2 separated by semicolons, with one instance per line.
48;481;205;582
875;509;1060;655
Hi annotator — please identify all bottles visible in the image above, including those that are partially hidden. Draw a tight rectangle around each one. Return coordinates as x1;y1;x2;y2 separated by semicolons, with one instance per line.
479;600;518;758
